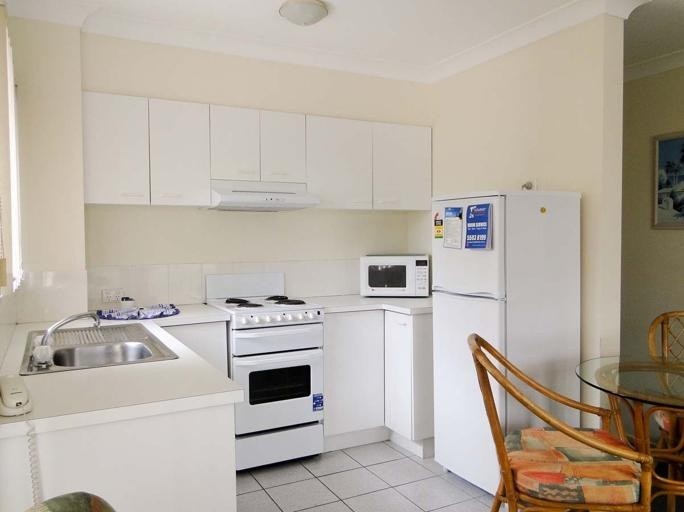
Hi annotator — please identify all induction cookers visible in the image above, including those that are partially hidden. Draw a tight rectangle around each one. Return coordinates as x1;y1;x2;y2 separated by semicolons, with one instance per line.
208;293;324;315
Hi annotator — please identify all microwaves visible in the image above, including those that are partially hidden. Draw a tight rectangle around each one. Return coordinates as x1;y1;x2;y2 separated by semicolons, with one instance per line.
358;254;431;300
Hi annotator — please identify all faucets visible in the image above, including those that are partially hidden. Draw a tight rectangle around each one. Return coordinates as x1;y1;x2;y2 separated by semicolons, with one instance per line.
41;311;101;343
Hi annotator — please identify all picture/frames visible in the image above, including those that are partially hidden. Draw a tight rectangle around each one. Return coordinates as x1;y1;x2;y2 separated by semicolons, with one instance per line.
652;130;684;231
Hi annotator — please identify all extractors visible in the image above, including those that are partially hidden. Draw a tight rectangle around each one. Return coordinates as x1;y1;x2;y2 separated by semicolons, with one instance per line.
197;179;319;215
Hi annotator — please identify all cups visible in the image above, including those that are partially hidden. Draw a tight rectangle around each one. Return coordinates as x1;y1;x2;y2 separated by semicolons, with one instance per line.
120;300;136;310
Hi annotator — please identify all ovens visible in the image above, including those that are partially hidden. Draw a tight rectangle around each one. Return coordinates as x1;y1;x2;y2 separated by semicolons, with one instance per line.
231;324;323;472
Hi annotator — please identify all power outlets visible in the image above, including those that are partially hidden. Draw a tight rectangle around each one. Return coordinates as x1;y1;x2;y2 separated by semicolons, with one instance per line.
102;290;123;303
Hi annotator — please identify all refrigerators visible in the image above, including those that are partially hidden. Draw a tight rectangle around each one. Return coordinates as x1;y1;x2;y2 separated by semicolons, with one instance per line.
428;188;584;512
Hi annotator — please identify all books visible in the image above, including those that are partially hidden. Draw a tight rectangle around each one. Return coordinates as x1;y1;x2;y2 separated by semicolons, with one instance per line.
464;203;493;250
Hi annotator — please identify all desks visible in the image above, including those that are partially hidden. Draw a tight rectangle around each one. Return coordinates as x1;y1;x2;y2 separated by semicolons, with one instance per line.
574;355;684;511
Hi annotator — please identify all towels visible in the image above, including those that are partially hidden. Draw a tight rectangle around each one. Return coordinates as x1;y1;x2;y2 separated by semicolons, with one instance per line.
97;304;179;320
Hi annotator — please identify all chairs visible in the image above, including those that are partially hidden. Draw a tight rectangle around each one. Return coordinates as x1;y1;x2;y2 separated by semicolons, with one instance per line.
648;310;684;473
468;333;654;511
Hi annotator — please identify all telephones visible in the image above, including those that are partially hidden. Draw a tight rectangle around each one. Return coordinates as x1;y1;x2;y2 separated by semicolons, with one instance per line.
0;374;32;417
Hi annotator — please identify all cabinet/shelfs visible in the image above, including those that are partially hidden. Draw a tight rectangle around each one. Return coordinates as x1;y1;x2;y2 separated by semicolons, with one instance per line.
385;305;435;459
306;115;433;211
322;305;390;453
210;104;306;183
82;92;211;208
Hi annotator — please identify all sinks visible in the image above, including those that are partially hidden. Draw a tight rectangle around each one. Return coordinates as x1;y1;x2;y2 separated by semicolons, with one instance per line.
19;323;180;374
